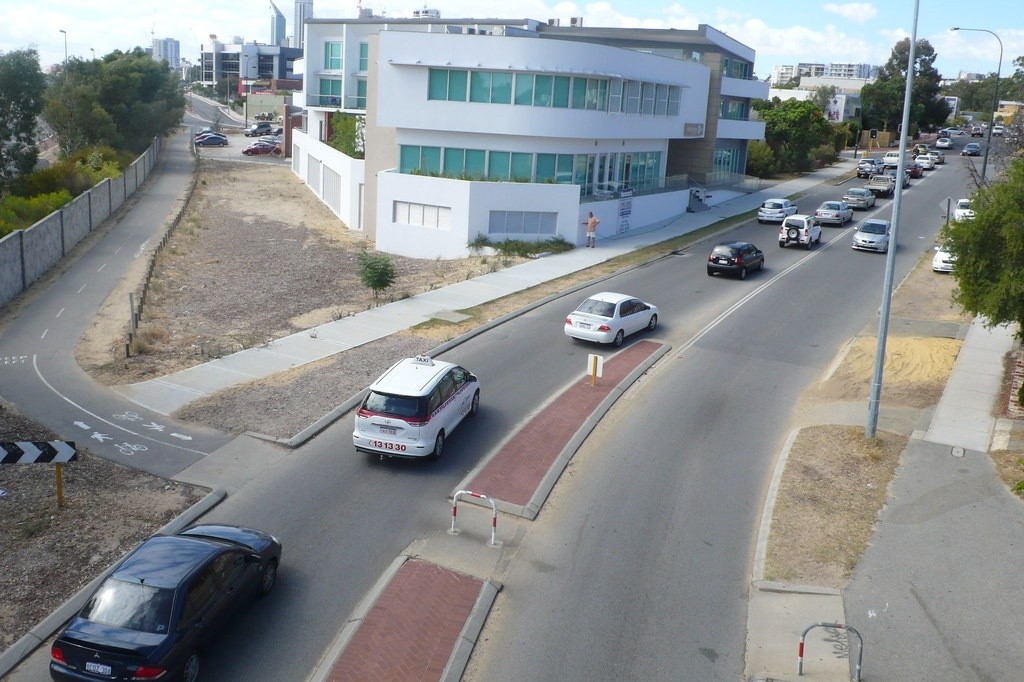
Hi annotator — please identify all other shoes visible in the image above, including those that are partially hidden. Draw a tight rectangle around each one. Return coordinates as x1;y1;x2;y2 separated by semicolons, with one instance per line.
592;246;594;248
586;245;589;247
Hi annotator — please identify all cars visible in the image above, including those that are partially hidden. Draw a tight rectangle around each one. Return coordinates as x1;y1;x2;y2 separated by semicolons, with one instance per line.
49;522;284;682
932;238;960;272
194;129;229;148
965;120;995;129
757;198;799;224
935;137;955;150
813;200;854;228
954;198;977;223
864;174;896;198
254;116;284;148
883;143;945;179
706;239;765;280
884;169;911;189
352;354;481;460
939;126;966;135
242;142;282;156
841;187;877;211
564;291;660;348
851;219;891;254
962;142;981;157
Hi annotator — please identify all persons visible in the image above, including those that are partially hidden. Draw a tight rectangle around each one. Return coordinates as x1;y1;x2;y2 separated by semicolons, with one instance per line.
580;211;601;248
912;145;920;156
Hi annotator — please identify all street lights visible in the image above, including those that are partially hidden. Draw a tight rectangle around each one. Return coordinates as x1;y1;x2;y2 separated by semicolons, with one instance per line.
212;69;231;117
244;56;265;128
949;27;1003;189
59;29;68;86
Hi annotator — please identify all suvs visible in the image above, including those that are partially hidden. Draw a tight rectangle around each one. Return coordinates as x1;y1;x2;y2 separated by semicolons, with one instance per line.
243;121;272;137
254;112;274;121
970;126;985;137
778;214;823;250
856;157;885;179
992;126;1004;136
936;130;951;140
